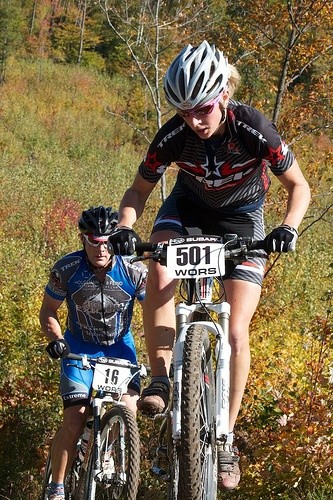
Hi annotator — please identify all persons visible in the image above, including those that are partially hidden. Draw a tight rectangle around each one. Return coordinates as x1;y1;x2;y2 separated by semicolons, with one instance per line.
39;204;149;500
107;39;313;493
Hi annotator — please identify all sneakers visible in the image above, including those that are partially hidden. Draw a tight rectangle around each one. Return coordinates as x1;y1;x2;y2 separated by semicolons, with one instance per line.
217;444;240;490
136;381;170;417
97;452;116;489
48;486;65;500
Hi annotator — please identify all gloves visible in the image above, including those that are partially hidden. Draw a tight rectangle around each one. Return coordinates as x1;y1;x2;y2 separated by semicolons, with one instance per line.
46;339;70;359
107;226;143;256
262;224;298;255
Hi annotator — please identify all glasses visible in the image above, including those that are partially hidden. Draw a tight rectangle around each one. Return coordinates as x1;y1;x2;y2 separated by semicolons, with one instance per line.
83;234;108;248
177;85;228;117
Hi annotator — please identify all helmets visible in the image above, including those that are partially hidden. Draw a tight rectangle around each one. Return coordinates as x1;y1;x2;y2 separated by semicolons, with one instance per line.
162;40;229;110
77;206;123;241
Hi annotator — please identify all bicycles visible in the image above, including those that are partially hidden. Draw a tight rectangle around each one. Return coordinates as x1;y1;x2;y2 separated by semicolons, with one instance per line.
41;352;151;500
129;236;270;499
